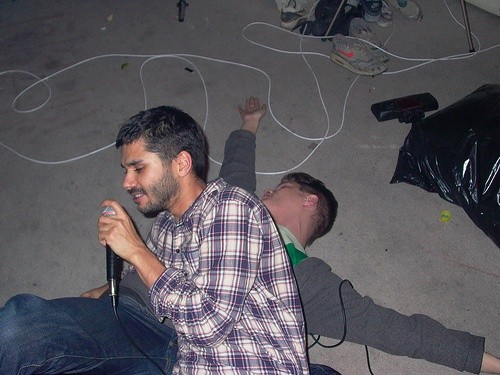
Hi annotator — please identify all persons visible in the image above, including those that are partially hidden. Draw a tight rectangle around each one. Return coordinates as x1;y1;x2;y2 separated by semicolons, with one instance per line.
79;105;310;375
0;96;500;375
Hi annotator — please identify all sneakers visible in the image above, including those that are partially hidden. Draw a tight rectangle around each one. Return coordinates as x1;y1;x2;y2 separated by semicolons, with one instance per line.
280;0;420;38
348;18;390;63
329;34;388;76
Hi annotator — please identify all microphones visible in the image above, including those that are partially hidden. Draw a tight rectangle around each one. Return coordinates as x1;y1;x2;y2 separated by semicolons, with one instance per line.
103;205;122;307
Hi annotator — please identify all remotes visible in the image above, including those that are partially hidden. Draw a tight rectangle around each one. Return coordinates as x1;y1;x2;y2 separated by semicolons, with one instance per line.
371;92;439;122
